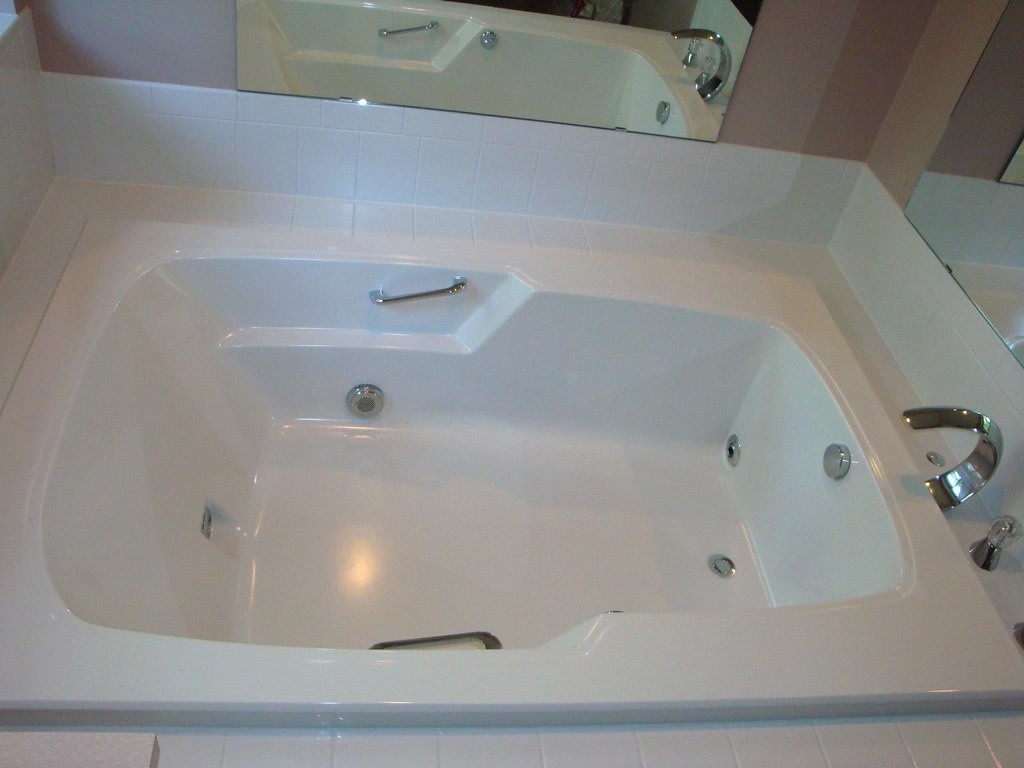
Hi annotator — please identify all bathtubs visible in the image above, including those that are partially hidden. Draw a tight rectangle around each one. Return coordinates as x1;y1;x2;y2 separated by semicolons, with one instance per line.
0;217;1024;722
237;1;720;145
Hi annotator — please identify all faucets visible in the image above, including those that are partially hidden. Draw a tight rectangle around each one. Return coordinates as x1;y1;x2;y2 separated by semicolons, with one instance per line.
902;407;1003;513
671;29;732;103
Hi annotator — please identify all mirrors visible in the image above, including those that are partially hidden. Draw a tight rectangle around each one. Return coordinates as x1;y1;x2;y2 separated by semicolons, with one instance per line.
901;0;1024;367
236;0;763;144
